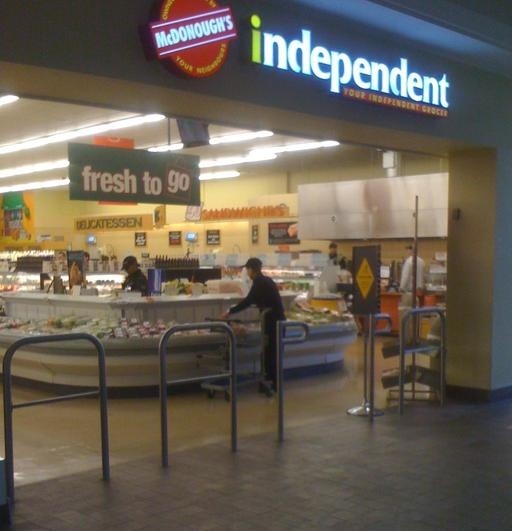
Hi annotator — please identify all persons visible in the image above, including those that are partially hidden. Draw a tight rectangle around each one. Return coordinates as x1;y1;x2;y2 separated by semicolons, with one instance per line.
328;243;346;269
220;258;286;392
120;256;147;292
399;246;425;338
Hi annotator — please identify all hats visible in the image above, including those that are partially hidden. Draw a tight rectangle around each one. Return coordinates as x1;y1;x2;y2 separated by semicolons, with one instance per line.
121;257;136;270
240;258;261;267
